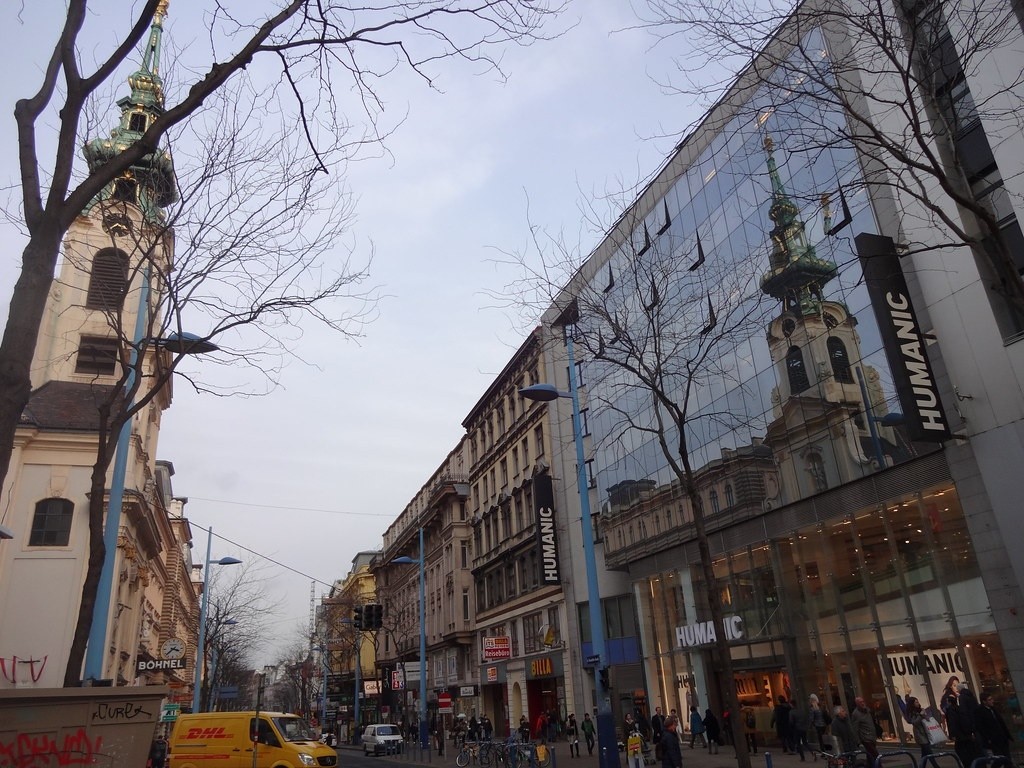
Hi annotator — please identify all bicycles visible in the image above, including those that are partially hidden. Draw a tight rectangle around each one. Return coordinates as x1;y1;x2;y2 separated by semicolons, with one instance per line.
812;748;862;768
456;741;551;768
454;727;465;749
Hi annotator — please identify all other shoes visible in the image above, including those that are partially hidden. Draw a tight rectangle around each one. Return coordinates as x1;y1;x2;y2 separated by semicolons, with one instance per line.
754;753;758;757
789;751;796;754
703;745;707;748
812;752;818;761
800;757;805;762
782;750;787;753
689;745;694;748
747;752;752;756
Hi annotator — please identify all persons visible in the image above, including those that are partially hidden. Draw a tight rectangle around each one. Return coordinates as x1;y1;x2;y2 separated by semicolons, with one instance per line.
311;690;1024;768
893;673;960;724
149;734;169;768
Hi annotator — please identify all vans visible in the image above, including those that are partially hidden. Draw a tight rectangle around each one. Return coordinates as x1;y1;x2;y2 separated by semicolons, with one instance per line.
361;724;404;757
164;710;338;768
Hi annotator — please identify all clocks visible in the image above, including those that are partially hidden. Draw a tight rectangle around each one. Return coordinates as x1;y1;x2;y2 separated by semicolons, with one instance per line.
103;212;133;238
161;637;186;660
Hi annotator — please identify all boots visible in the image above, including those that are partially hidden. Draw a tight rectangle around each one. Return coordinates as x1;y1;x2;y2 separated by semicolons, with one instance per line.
707;743;712;755
575;743;580;757
570;744;574;758
714;743;718;754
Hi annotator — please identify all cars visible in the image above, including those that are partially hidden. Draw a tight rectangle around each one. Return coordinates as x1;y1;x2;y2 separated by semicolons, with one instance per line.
319;734;338;747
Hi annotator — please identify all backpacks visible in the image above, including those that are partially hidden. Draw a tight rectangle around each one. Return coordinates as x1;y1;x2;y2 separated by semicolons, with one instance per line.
744;714;755;729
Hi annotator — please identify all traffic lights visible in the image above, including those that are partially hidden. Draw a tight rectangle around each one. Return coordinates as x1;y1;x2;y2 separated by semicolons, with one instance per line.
355;604;383;631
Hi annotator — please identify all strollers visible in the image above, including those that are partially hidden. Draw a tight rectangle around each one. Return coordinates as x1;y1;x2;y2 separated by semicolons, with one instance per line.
629;730;656;765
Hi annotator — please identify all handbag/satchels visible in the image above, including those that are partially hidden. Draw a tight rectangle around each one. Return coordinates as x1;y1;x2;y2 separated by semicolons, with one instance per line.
921;717;948;746
821;706;832;725
871;714;883;738
718;736;725;746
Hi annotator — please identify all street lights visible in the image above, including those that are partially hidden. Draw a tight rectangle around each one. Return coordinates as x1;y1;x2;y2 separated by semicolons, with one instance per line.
192;558;242;713
517;383;622;768
391;556;430;749
339;618;360;745
81;330;219;687
208;619;239;712
856;366;904;469
313;646;327;734
